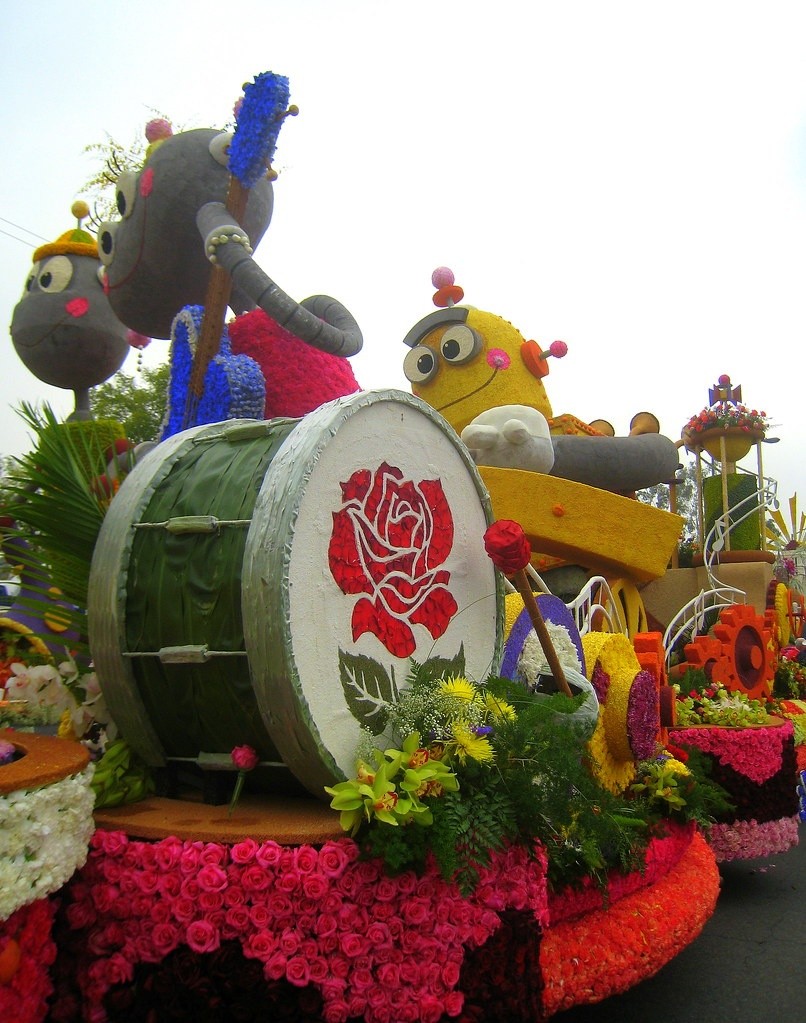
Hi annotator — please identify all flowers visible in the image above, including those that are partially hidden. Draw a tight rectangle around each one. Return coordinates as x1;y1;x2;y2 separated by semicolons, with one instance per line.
81;104;219;237
227;72;289;185
782;540;806;581
155;302;363;441
684;407;772;436
0;611;806;1023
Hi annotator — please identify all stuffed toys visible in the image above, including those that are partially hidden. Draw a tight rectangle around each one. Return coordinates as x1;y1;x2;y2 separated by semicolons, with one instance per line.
0;71;680;707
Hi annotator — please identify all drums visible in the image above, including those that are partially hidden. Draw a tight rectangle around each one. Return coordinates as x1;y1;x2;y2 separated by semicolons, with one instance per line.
89;386;502;804
